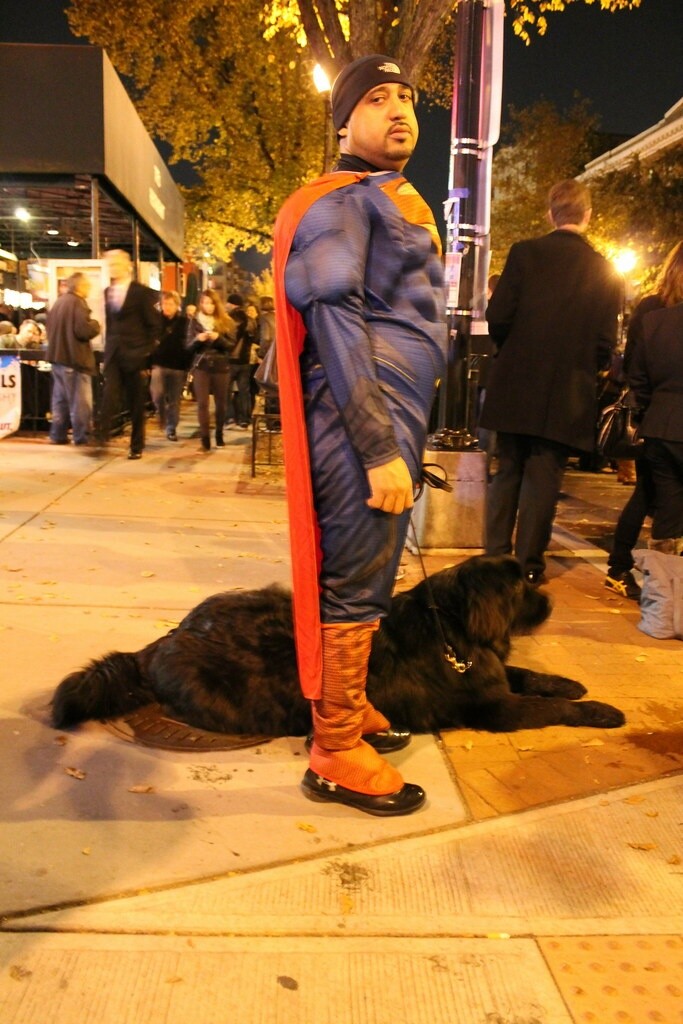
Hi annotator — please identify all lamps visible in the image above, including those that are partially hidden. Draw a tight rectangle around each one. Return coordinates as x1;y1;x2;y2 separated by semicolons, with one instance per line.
67;239;80;246
47;226;60;235
32;299;49;310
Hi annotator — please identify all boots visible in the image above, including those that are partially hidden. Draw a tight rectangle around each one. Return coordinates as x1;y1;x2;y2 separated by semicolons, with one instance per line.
615;460;637;483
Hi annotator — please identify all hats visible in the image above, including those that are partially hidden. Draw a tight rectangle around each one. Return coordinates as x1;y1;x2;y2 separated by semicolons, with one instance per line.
331;54;414;133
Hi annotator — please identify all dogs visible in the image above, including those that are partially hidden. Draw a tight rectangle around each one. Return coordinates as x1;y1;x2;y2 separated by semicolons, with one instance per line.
47;555;625;733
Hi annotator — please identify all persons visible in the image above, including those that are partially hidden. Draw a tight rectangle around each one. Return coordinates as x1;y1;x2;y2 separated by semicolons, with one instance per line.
605;240;683;641
0;312;47;349
271;53;452;816
149;290;276;451
44;273;101;445
76;250;162;460
483;178;625;587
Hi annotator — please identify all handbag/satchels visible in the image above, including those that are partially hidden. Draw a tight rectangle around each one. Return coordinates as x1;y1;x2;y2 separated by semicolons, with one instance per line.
632;548;683;639
597;386;645;462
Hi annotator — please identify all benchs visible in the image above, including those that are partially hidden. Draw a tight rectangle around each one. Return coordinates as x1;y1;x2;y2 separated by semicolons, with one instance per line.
248;391;283;479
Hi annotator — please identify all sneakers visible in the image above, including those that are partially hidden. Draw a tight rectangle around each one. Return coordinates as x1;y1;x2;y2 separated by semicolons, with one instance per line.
604;568;641;602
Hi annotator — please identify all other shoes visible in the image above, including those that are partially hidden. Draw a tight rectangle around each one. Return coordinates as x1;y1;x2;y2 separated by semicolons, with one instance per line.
48;439;71;445
167;433;178;442
129;450;142;459
238;421;248;429
523;571;546;588
225;418;234;429
599;465;614;472
201;434;211;450
216;432;224;446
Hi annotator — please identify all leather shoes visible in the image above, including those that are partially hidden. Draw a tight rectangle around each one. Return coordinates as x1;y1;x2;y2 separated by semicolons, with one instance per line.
305;728;409;754
300;767;425;817
